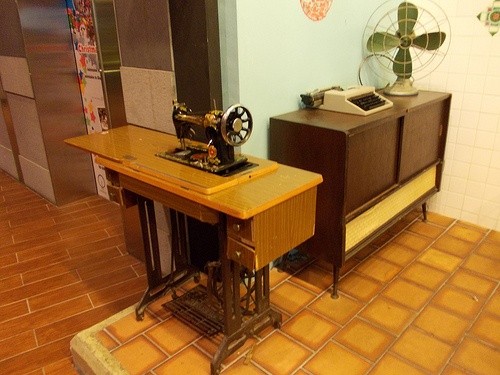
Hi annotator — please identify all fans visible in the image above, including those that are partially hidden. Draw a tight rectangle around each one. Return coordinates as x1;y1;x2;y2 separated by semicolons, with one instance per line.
361;2;452;96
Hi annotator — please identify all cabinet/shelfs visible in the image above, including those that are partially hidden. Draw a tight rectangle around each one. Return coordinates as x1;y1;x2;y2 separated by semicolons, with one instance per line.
270;85;453;302
